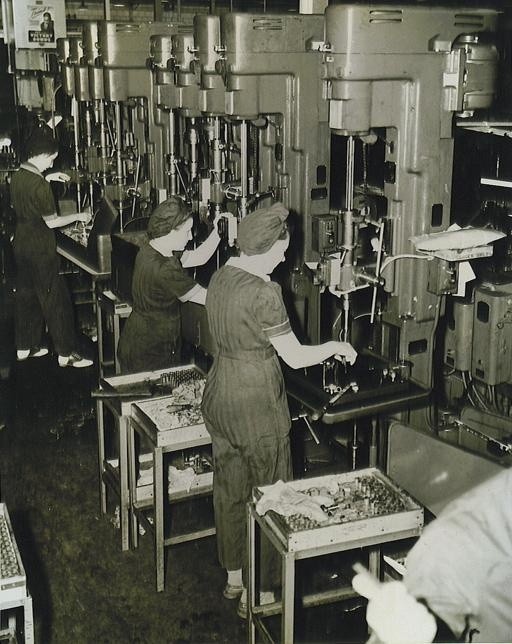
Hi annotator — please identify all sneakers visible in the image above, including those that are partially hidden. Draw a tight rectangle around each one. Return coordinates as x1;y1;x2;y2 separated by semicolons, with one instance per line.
58;352;94;368
222;584;255;619
16;346;49;360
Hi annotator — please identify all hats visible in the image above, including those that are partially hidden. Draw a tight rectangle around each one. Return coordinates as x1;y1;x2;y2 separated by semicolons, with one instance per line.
147;197;192;240
25;126;56;153
238;202;290;256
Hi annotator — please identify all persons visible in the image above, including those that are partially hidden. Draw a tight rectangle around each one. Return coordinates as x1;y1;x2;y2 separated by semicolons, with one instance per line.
112;196;237;457
364;464;512;642
9;131;97;367
201;200;358;619
